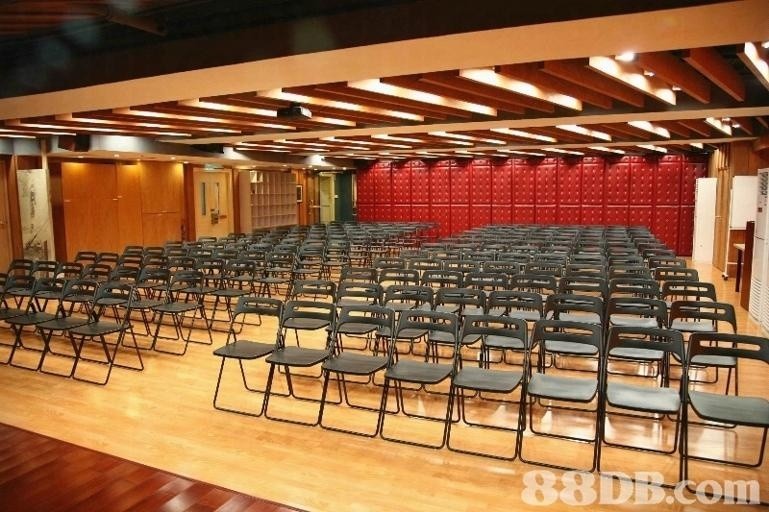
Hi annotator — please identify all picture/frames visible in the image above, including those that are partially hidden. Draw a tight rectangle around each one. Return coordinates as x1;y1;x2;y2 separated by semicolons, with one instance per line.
297;184;303;203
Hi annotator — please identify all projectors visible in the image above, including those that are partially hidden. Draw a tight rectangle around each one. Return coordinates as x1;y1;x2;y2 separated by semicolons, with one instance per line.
277;106;311;121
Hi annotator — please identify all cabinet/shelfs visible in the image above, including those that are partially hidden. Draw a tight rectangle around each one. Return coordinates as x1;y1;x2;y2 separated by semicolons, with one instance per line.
238;168;298;235
